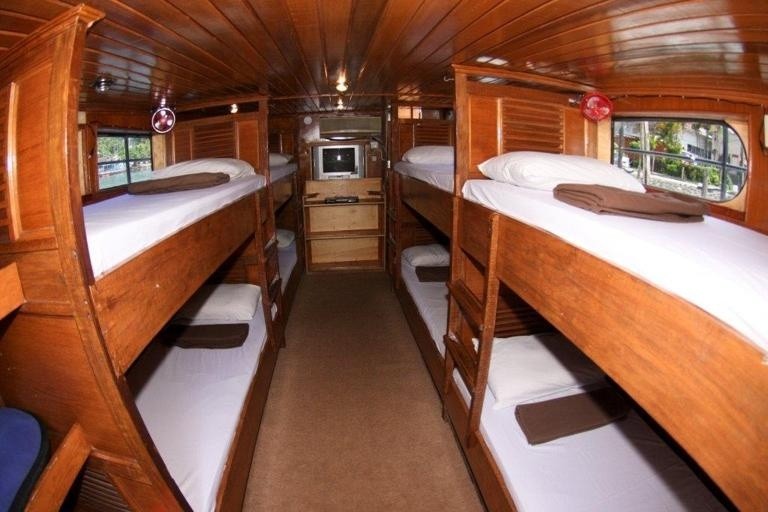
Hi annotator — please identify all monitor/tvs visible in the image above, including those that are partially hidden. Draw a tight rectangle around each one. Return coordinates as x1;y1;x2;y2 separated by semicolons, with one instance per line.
313;144;364;181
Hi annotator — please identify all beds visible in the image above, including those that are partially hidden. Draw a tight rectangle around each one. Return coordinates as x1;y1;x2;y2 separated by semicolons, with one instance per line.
385;61;768;510
0;4;311;512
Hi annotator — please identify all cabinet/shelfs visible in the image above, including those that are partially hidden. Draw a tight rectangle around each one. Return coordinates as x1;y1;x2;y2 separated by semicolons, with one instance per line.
299;178;384;271
